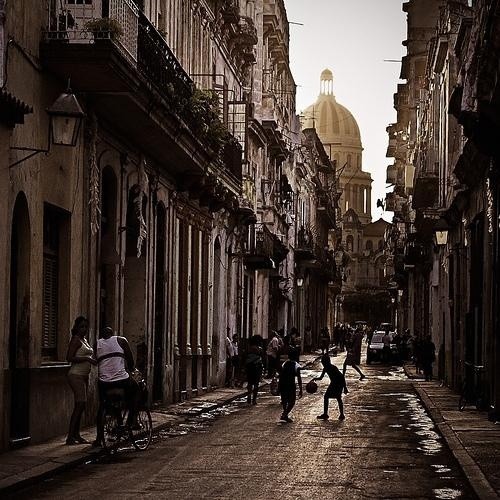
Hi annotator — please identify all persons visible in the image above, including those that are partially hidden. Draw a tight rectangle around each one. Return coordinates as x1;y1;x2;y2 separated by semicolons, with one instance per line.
93;327;142;446
310;354;348;420
66;316;98;446
278;348;303;423
227;321;445;405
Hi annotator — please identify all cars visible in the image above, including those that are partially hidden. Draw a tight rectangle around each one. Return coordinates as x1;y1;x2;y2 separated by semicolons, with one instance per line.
366;330;399;365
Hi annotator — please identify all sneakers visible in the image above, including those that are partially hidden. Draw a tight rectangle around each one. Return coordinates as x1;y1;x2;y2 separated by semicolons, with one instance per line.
318;413;329;419
339;415;345;419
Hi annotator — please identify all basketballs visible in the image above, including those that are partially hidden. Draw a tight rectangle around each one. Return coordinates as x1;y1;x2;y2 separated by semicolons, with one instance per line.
306;383;317;393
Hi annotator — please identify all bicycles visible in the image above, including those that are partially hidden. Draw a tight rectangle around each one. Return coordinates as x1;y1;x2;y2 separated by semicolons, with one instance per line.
457;360;488;412
98;402;153;457
